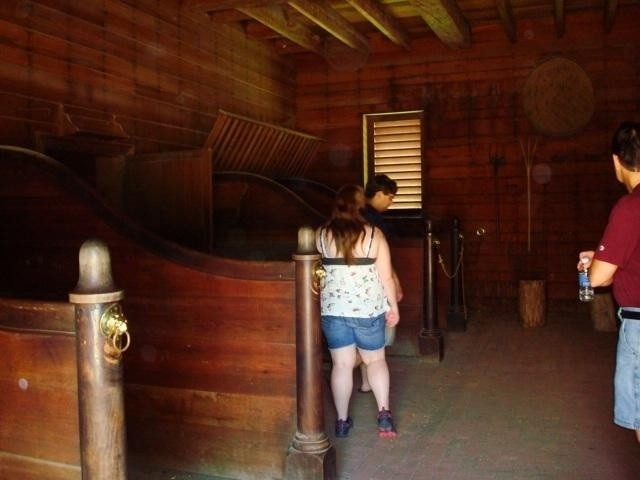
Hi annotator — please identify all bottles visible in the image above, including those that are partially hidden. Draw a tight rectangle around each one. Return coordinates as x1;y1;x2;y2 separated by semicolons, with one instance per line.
577;256;595;301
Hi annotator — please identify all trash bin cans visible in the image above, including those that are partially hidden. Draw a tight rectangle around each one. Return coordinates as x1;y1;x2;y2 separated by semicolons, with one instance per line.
591;288;617;333
519;280;547;328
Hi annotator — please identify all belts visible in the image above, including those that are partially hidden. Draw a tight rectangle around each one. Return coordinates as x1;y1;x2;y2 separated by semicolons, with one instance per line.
619;308;640;320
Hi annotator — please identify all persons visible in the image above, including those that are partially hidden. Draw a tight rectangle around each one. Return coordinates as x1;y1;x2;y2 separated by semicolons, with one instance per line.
576;119;640;448
350;174;405;393
314;182;401;440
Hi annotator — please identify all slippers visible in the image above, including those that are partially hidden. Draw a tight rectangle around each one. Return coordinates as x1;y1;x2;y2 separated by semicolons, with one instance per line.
358;388;371;394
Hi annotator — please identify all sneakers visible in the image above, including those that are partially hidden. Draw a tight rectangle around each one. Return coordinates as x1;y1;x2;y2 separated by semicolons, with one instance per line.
375;406;398;438
334;417;354;438
